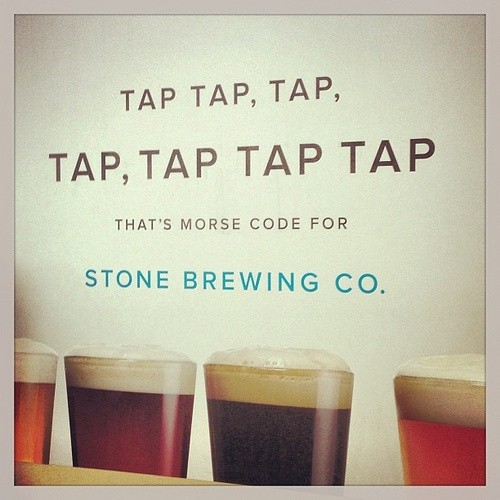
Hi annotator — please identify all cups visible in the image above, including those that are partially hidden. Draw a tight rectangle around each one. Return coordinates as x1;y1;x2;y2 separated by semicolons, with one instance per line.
64;355;197;479
15;351;58;463
203;364;354;485
392;376;486;486
